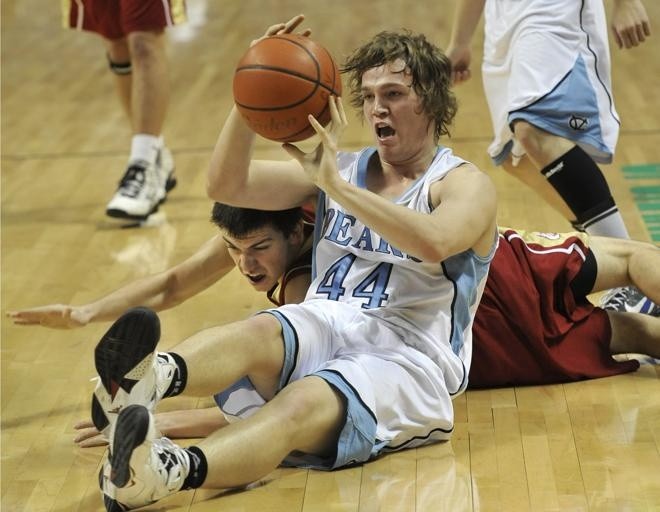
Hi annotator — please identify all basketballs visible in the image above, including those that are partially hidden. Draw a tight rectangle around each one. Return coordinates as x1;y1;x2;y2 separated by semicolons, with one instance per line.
233;33;342;142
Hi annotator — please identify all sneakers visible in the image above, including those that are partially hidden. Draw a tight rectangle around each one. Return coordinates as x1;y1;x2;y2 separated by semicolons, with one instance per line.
599;285;660;316
91;305;191;512
105;146;177;220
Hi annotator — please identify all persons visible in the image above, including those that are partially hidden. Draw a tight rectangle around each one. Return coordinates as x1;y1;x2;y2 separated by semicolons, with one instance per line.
437;0;659;319
3;195;660;452
58;0;196;222
88;11;502;512
